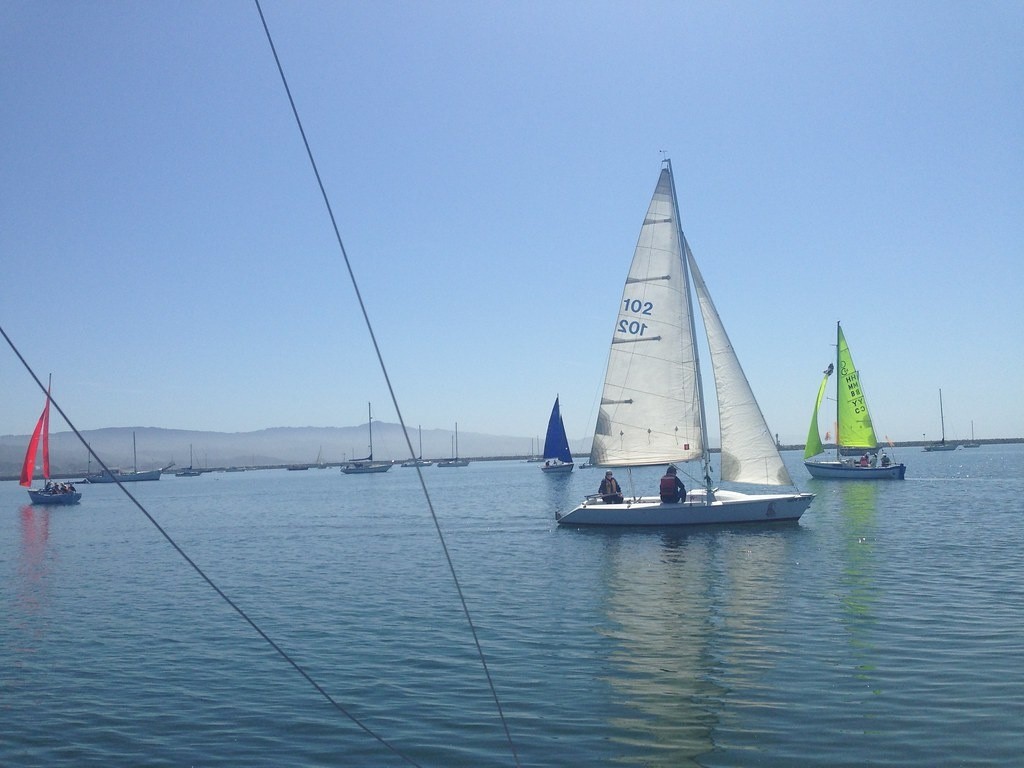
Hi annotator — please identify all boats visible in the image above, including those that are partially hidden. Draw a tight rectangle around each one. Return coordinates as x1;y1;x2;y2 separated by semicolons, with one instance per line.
287;464;309;470
226;465;248;472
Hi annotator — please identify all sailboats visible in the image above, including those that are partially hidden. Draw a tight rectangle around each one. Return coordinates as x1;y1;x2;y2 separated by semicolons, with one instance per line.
541;392;575;474
340;403;393;473
552;154;816;527
923;390;961;451
964;420;981;447
801;321;905;482
437;422;470;467
401;425;432;467
316;446;328;469
526;436;545;462
82;432;175;484
19;372;81;503
175;443;202;477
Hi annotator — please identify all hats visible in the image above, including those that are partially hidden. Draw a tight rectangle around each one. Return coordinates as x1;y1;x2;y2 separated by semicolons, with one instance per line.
607;473;613;475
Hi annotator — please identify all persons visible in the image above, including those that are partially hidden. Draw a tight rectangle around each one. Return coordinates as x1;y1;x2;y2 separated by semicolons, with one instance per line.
860;453;891;467
546;460;558;466
45;481;76;495
598;471;624;504
660;466;686;503
